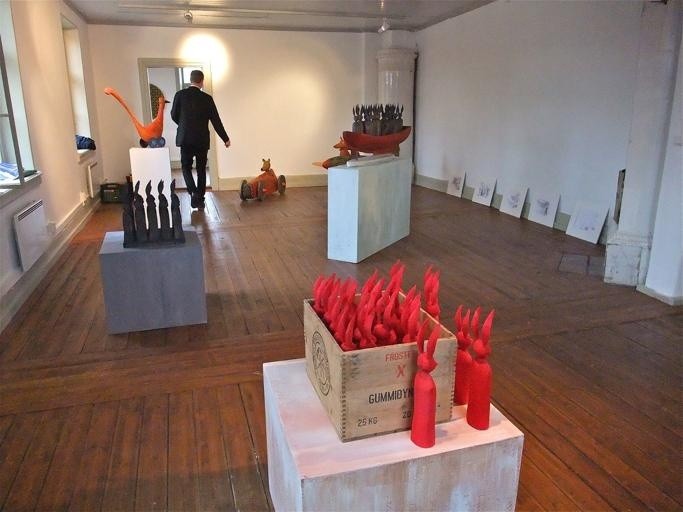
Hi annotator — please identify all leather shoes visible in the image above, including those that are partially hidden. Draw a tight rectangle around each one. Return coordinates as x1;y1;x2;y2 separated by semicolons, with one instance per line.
189;192;206;210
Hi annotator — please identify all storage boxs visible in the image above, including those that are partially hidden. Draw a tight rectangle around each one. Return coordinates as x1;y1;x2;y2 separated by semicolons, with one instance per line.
303;287;457;442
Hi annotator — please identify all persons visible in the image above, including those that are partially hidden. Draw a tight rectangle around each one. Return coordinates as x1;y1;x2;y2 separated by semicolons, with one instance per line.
170;70;230;208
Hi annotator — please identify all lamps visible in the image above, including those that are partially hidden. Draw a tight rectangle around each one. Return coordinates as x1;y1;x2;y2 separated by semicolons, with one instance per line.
183;7;391;34
